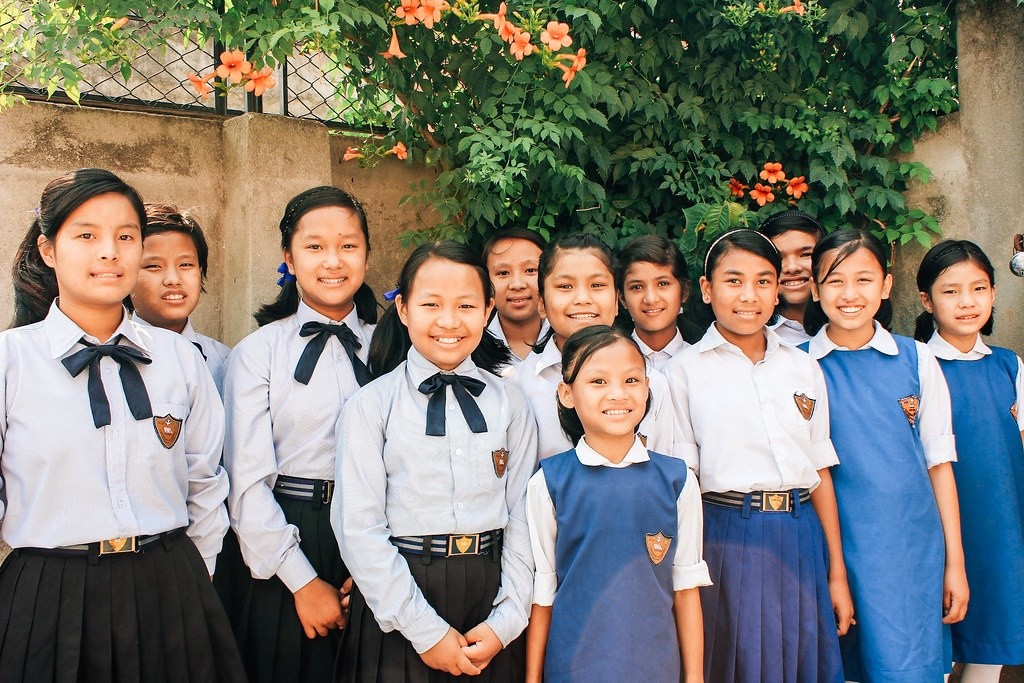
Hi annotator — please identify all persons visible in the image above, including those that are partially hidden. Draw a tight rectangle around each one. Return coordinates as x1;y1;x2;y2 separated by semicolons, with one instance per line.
0;167;1024;683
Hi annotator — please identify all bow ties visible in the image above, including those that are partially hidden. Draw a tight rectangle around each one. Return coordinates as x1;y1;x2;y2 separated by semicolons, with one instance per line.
294;321;374;387
60;334;152;428
418;372;486;435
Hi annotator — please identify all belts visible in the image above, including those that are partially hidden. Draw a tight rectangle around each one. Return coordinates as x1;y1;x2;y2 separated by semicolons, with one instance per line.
389;530;502;557
272;476;335;505
39;529;178;558
700;490;811;513
